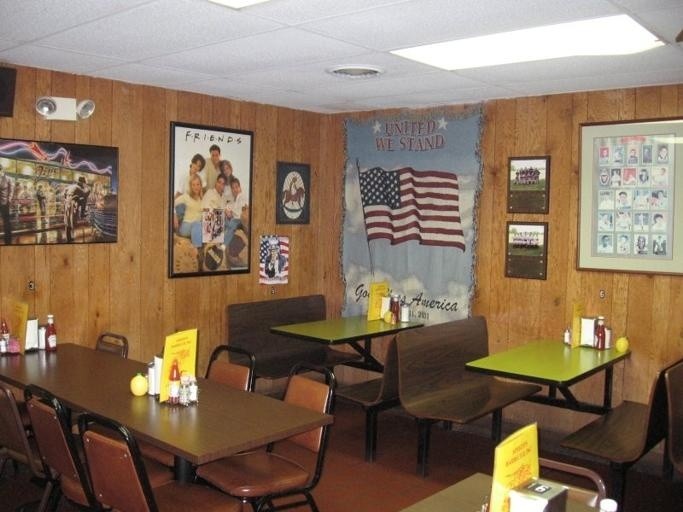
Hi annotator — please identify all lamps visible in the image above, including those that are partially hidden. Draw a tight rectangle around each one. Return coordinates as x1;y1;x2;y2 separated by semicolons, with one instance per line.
34;94;96;121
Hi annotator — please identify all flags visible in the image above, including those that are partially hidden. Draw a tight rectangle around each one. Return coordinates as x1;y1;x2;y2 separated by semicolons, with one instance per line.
358;167;465;252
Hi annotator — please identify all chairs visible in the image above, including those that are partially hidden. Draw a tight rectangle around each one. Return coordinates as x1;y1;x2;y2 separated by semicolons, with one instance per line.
535;455;607;509
77;411;245;511
94;331;128;358
195;360;338;510
0;384;87;511
22;383;175;511
137;345;257;466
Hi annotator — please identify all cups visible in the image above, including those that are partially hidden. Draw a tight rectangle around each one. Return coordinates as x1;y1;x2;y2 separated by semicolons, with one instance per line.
604;328;611;349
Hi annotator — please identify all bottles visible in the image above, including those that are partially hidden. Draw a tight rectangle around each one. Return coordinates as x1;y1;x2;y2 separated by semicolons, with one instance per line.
0;313;57;356
128;350;199;406
615;336;630;352
128;394;199;442
0;351;59;376
379;294;411;325
594;314;605;350
563;329;569;343
562;344;612;373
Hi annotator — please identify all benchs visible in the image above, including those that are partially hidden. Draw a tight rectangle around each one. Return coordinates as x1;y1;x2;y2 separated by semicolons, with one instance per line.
329;333;400;466
227;293;362;380
398;314;542;477
663;362;682;476
562;357;682;466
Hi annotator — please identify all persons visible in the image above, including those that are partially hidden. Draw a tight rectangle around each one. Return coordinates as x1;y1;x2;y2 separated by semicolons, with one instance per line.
513;165;541;186
36;184;46;216
174;142;249;272
63;181;91;242
510;231;539;250
0;163;14;245
598;145;668;256
264;244;286;280
18;185;32;215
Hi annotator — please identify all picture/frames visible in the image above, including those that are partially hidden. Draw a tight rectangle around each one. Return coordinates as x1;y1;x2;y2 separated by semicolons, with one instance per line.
0;136;118;244
505;155;551;213
274;160;310;226
575;115;682;279
167;122;251;277
502;220;549;279
